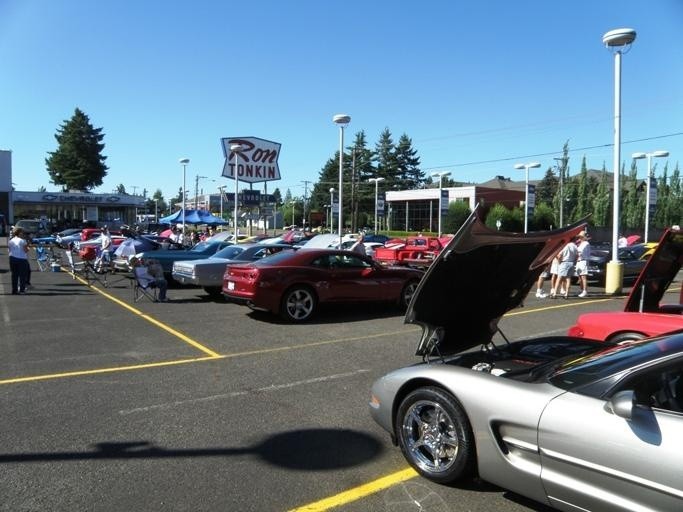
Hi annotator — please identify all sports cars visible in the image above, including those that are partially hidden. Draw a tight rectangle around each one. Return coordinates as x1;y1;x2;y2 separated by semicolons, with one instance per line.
219;247;426;323
366;203;682;511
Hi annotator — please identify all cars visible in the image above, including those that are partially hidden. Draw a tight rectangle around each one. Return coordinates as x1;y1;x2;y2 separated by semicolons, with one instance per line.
209;224;455;265
539;234;664;286
120;223;231;284
572;224;682;358
10;218;132;263
112;236;188;275
169;240;298;300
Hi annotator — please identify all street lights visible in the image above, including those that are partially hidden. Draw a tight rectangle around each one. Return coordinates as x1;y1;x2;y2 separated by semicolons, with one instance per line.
289;201;296;229
178;158;188;237
217;185;226;233
602;27;637;299
367;177;384;235
332;114;350;236
322;204;331;228
326;188;335;234
553;156;568;229
514;163;540;234
630;149;670;246
153;198;158;223
432;171;452;239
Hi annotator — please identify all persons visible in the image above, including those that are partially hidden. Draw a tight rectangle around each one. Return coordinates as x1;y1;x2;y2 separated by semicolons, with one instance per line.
535;231;591;297
350;233;367;260
93;225;110;271
97;228;114;274
618;233;627;248
170;223;216;246
130;257;170;303
9;226;34;294
418;232;423;237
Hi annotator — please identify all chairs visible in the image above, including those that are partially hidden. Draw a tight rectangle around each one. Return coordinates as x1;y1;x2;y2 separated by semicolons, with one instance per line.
33;242;173;308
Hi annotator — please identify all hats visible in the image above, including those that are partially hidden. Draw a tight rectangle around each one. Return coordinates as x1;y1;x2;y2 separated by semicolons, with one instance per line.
127;256;141;268
575;230;589;241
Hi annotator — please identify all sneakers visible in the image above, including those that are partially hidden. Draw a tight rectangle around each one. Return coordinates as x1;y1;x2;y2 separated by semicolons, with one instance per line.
12;283;34;295
535;286;589;300
158;297;171;303
